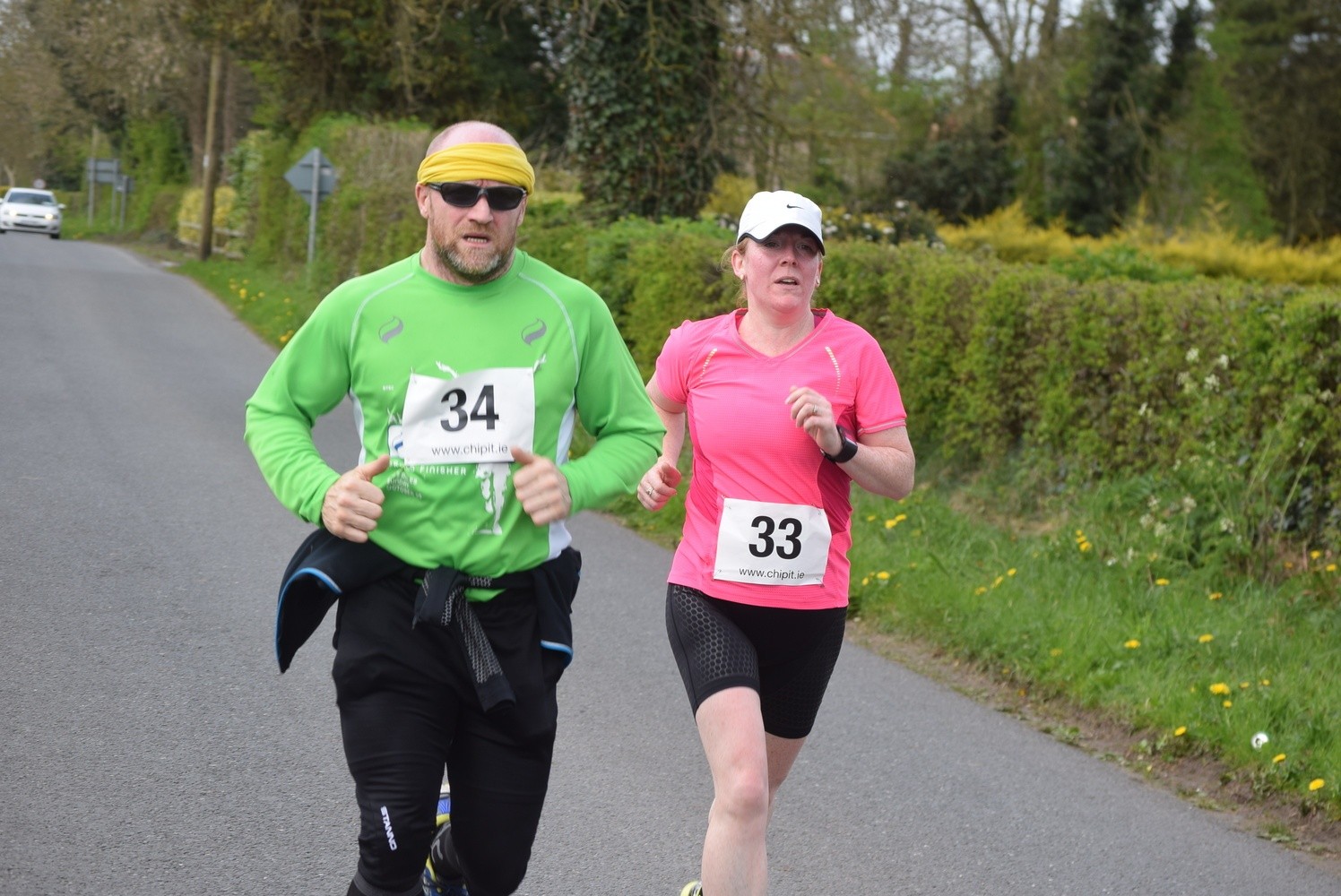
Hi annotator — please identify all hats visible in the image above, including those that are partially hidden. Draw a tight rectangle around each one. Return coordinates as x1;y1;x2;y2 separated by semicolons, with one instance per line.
735;190;828;256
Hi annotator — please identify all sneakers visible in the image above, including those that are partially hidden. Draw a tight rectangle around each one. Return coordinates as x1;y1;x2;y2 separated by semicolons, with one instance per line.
421;784;473;896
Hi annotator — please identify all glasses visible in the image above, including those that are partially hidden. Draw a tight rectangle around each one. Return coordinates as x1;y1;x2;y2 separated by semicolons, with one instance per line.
425;181;527;212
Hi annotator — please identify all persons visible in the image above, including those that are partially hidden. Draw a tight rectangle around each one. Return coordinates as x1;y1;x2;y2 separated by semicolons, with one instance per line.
634;190;919;896
240;118;671;896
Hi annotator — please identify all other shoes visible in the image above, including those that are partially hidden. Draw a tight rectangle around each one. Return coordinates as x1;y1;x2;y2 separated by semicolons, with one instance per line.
680;880;702;896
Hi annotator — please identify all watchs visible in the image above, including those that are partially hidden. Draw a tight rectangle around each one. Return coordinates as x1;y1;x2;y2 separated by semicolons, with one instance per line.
820;425;859;463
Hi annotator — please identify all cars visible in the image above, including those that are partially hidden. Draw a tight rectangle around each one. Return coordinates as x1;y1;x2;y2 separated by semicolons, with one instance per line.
0;188;67;239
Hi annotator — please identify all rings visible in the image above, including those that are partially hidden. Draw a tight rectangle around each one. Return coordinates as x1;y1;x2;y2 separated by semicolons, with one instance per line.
645;487;653;495
813;404;818;415
